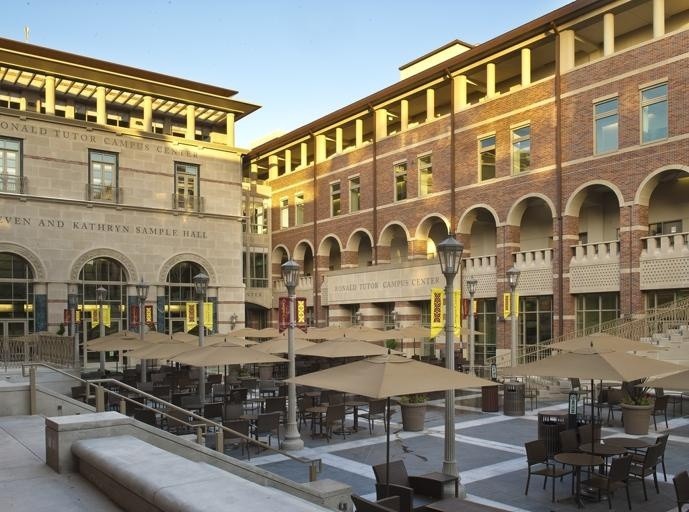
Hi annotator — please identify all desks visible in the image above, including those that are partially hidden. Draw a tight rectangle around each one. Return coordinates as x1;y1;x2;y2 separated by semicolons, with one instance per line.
306;406;328;438
424;498;512;511
603;437;648;449
580;443;627;478
420;472;458;498
342;402;367;431
552;451;603;508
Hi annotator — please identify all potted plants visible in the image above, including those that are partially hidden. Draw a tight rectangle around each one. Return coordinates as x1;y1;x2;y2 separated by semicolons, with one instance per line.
399;395;428;432
619;396;652;435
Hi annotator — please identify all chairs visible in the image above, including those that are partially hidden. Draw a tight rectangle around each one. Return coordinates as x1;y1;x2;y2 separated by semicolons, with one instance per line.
631;433;668;483
671;470;688;512
583;454;632;511
242;378;256;399
231;389;249;415
559;429;579;481
260;380;276;396
72;386;85;403
411;355;445;366
524;438;571;501
204;374;222;394
251;413;281;454
133;407;165;428
373;459;441;511
629;442;662;501
569;377;581;391
108;391;121;413
297;395;319;433
222;417;251;461
181;397;203;415
203;403;223;431
153;387;168;409
526;388;537;411
585;388;611;421
212;383;232;403
320;404;346;442
350;494;402;512
651;395;668;430
265;396;287;431
329;392;345;404
358;399;387;435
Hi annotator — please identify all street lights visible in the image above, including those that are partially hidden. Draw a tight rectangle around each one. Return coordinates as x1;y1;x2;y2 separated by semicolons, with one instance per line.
505;267;521;385
280;260;300;458
436;236;464;490
93;287;107;385
191;272;207;451
135;283;149;418
466;278;478;391
67;293;78;370
